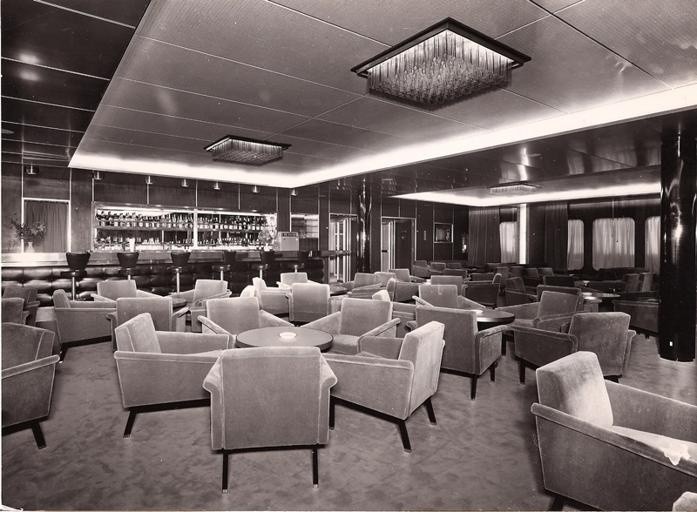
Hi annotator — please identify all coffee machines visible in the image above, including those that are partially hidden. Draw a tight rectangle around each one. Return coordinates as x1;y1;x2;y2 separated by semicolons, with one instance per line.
276;231;301;252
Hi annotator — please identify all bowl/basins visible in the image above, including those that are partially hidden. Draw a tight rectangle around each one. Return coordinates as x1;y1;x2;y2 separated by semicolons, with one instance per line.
279;331;297;341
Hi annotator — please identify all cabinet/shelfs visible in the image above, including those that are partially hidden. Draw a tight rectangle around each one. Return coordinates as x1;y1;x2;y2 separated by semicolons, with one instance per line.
94;209;277;248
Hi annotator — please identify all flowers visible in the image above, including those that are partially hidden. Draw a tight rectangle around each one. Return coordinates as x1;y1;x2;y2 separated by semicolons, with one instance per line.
9;216;48;245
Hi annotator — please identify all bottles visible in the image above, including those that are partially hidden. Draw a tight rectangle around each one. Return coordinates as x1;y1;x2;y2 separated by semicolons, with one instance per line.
94;210;272;253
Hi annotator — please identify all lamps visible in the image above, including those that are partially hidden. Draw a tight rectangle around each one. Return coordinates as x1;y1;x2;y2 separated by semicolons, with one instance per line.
203;135;292;166
350;16;533;113
93;171;104;181
212;182;222;190
145;175;154;185
253;186;260;193
180;178;190;188
486;180;544;195
24;162;39;176
290;188;297;196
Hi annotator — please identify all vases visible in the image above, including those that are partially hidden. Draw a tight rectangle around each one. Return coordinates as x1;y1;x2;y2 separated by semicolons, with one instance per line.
24;241;35;253
